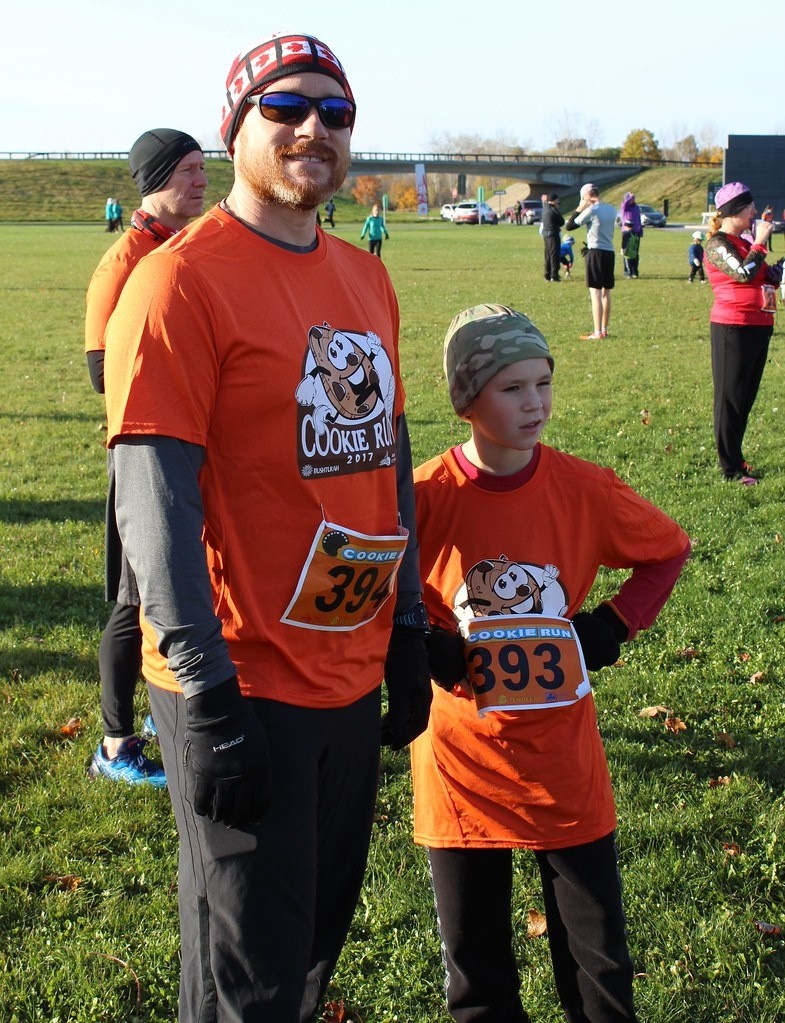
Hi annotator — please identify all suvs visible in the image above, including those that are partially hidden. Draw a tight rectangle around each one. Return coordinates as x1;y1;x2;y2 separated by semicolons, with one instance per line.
439;204;457;221
453;201;499;224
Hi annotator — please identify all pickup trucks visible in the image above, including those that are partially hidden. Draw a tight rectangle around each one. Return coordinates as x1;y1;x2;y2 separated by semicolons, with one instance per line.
504;200;542;226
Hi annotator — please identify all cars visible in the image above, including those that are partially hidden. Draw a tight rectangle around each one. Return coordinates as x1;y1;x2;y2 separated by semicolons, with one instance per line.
617;204;667;228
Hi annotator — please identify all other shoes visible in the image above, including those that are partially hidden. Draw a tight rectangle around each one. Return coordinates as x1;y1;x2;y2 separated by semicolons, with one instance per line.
736;474;758;486
580;332;607;340
321;1001;360;1023
742;462;758;476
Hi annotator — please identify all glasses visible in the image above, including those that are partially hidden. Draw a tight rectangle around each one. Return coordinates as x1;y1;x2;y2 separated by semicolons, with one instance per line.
245;90;356;130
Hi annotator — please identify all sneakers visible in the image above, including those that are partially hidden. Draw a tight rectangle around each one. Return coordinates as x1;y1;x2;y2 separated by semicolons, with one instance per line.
93;734;172;789
144;715;155;738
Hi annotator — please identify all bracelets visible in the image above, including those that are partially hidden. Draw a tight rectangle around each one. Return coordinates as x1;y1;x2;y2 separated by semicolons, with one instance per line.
750;243;767;257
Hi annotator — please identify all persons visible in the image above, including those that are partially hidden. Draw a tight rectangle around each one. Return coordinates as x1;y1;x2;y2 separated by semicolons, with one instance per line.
704;183;785;484
406;304;692;1023
105;27;435;1021
537;182;643;283
103;197;126;234
361;203;390;259
761;204;774;252
323;198;336;228
83;130;207;788
514;200;522;226
565;183;617;340
687;229;707;284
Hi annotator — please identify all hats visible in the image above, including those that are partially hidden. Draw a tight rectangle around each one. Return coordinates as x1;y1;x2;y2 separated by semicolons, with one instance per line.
114;198;119;202
129;129;200;197
625;218;634;225
714;182;752;210
107;198;113;205
692;231;704;239
579;183;598;198
547;192;558;201
624;192;633;198
563;234;575;244
443;303;554;412
219;29;355;162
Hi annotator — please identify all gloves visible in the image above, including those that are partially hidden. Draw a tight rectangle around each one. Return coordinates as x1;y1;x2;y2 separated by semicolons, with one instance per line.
183;676;272;832
428;625;466;693
570;603;628;671
368;641;432;751
